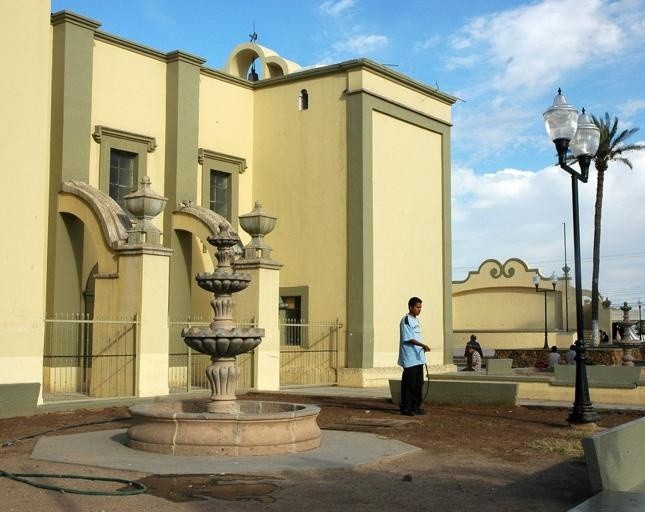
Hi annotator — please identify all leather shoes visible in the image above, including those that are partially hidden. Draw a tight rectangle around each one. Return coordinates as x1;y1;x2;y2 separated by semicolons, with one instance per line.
414;408;427;415
400;410;415;416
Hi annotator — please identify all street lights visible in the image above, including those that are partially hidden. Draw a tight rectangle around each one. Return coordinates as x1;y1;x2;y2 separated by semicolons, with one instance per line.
544;88;602;424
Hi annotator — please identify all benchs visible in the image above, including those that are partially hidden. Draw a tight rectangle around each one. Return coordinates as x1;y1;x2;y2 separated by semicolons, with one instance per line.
549;363;641;389
477;358;514;376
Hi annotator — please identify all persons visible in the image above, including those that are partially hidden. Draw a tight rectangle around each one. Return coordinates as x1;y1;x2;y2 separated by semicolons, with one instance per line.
461;344;484;372
566;345;577;364
574;339;593;364
600;329;605;339
397;296;430;415
464;334;483;365
602;332;609;342
546;345;562;368
